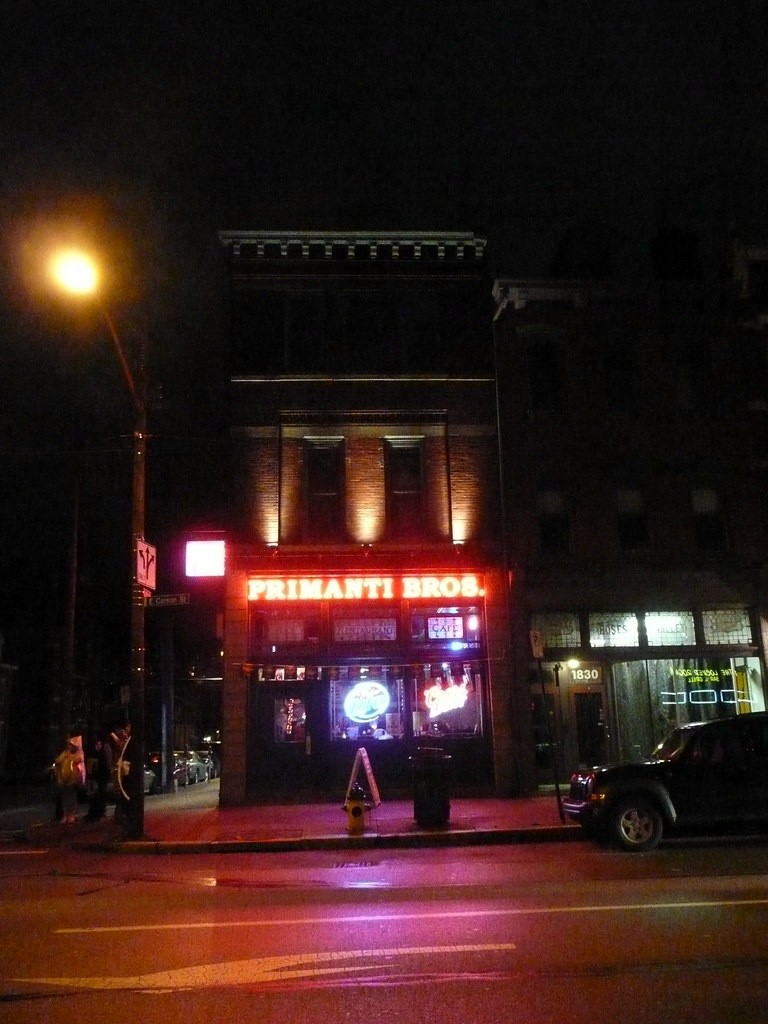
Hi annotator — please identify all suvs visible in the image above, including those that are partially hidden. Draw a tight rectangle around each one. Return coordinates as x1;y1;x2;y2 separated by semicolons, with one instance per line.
559;709;768;851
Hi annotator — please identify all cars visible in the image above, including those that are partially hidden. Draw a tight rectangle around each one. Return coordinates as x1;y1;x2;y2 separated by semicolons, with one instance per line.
142;747;221;794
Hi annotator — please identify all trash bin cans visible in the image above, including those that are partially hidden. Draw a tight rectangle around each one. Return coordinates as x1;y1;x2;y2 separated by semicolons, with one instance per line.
408;747;452;827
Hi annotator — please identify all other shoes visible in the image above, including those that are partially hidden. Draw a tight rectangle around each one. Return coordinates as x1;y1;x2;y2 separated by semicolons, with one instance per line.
61;816;67;825
67;816;74;824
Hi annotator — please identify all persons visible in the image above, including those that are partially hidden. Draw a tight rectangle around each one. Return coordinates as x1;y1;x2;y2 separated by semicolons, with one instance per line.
53;724;139;826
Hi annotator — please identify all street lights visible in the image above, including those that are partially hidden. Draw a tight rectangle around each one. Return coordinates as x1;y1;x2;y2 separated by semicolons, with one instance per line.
43;242;149;841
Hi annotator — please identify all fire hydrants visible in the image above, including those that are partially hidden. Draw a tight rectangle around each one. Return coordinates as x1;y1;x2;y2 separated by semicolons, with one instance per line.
340;781;372;836
